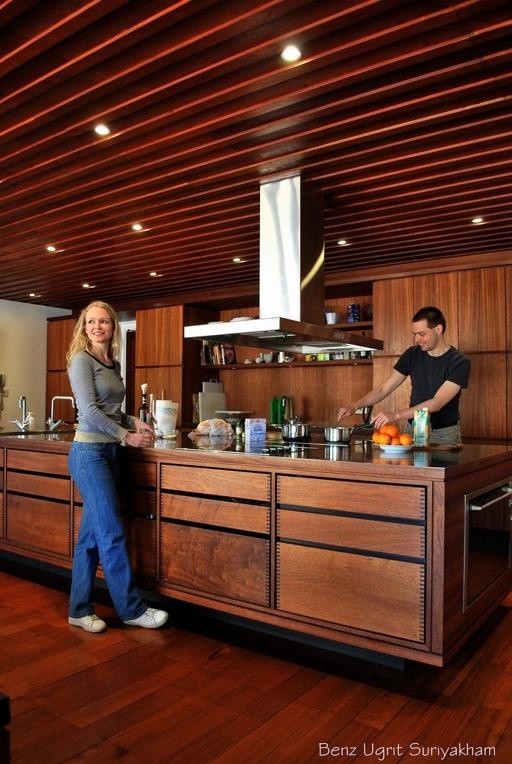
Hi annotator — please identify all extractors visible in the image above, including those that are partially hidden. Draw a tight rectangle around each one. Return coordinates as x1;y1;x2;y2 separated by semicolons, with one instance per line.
183;176;383;354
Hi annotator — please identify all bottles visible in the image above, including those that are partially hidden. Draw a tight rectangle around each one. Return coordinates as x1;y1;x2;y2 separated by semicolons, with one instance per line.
138;393;147;423
270;395;279;424
236;421;242;440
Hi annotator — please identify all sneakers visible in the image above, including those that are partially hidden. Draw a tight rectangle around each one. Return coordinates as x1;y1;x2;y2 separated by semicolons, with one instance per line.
123;606;169;630
67;613;108;633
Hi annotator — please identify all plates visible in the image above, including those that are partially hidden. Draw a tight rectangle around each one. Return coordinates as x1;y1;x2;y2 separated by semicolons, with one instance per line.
373;443;414;454
379;453;413;461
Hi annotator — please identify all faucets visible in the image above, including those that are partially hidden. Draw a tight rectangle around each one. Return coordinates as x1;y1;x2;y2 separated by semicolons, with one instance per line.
48;396;75;431
10;395;30;432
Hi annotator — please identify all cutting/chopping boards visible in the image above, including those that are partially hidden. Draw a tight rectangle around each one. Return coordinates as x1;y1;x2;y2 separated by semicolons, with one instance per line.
199;392;226;423
202;379;224;393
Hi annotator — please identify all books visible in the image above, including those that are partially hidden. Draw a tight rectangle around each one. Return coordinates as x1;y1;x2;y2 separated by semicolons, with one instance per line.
201;339;236;365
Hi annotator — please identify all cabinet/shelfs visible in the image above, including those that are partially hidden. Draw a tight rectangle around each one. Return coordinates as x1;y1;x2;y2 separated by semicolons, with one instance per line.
414;272;458;352
157;459;272;613
458;267;506;352
134;368;181;428
47;320;78;372
273;473;433;654
182;280;371;434
3;447;72;562
443;459;512;651
372;276;413;356
457;351;507;439
134;306;184;367
74;457;158;584
507;264;512;352
47;373;77;424
373;357;412;426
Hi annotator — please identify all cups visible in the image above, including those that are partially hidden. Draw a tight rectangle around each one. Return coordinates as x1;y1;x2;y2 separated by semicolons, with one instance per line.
325;313;336;325
155;438;176;449
155;400;172;418
160;403;177;430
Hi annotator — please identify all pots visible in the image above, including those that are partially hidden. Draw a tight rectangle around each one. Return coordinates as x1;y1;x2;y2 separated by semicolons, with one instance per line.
323;424;375;445
323;445;350;462
281;424;311;442
283;443;308;459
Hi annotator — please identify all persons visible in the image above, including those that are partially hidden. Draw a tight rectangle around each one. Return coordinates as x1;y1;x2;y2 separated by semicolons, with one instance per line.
66;301;170;633
337;307;470;444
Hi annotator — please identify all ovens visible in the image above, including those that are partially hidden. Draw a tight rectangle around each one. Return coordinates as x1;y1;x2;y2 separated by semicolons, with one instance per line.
462;474;512;615
